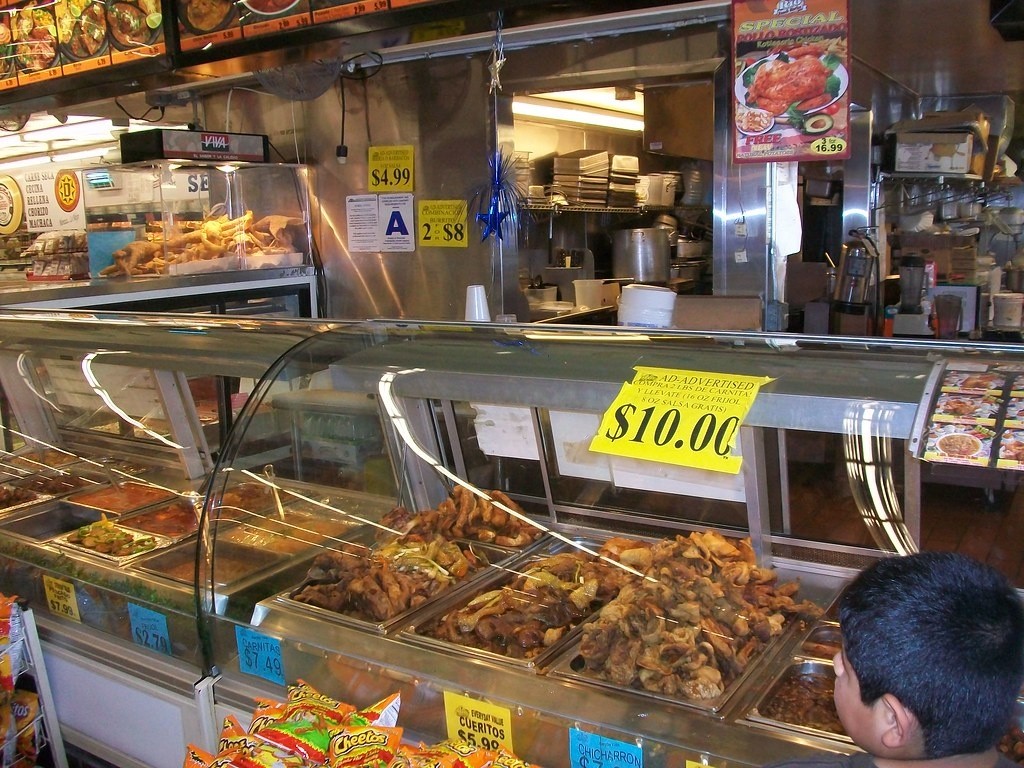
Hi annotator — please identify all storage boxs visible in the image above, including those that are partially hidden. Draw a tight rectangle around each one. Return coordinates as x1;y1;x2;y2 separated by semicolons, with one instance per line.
0;267;318;486
642;79;715;163
189;321;1024;768
949;235;979;272
82;158;318;278
640;174;678;206
894;132;973;173
896;233;954;276
5;299;473;765
669;293;763;332
927;280;982;334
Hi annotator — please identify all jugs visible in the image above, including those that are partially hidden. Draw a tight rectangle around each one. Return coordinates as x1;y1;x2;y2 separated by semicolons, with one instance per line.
933;294;963;339
896;252;930;314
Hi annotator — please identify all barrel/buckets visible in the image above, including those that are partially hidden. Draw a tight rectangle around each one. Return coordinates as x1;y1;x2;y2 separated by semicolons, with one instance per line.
992;293;1024;328
615;284;677;328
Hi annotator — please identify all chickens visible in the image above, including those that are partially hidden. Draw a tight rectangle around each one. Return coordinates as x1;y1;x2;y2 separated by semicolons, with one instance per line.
746;43;832;117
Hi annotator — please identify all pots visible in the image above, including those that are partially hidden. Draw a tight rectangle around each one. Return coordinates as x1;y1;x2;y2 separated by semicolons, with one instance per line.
606;227;678;286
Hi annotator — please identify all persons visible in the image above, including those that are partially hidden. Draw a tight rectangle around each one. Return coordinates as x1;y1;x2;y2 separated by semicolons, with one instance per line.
763;551;1024;768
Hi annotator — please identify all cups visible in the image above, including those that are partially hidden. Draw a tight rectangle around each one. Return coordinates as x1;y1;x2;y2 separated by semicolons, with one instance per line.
464;284;491;322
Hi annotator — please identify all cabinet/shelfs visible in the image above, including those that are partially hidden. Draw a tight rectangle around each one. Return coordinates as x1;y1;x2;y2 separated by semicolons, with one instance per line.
0;589;73;768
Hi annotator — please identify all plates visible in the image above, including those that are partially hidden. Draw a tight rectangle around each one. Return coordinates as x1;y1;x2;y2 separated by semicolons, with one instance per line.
734;52;849;116
234;1;301;24
735;108;774;135
60;0;108;62
0;55;15;81
175;1;236;37
14;7;60;74
935;433;983;456
108;19;163;53
